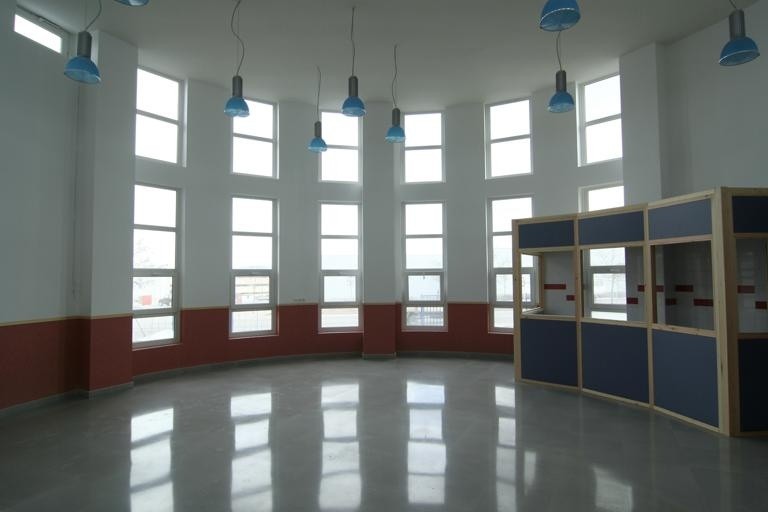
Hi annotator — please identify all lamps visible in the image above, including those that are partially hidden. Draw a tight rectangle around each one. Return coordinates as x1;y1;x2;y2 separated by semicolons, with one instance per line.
308;62;329;152
341;5;366;118
385;42;406;145
537;0;582;33
547;22;576;113
222;0;251;119
63;0;101;85
717;0;761;67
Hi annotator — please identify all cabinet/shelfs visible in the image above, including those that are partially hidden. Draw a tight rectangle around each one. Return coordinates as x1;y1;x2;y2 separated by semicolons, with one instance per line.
511;186;768;439
